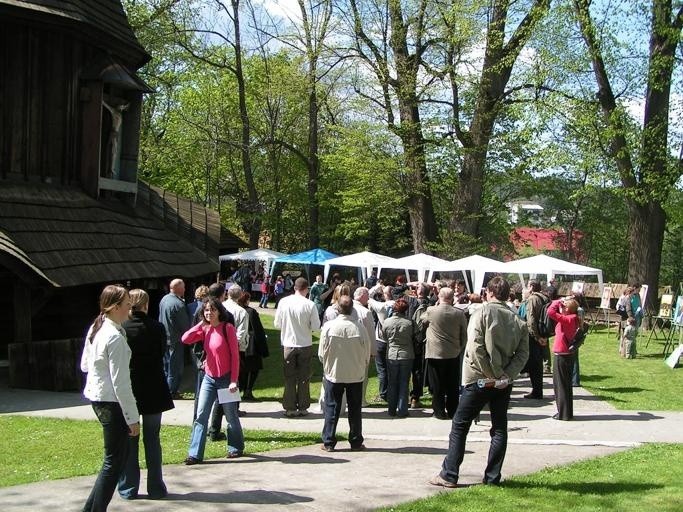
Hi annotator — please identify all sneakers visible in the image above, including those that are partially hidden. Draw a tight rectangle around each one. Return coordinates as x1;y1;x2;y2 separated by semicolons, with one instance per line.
210;432;226;442
226;451;244;458
184;456;203;465
241;395;261;403
351;444;366;451
517;372;527;378
320;443;334;452
283;407;309;418
543;364;550;373
238;410;246;417
429;474;456;487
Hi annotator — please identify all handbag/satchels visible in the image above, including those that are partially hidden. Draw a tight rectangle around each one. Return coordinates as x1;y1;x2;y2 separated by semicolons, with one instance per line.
616;304;628;318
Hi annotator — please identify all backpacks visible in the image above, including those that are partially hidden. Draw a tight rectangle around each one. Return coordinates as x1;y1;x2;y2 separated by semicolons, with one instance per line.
564;316;586;352
533;293;555;337
411;298;433;343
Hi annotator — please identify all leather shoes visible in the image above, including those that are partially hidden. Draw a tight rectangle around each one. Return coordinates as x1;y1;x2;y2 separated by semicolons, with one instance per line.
552;414;569;421
388;398;452;419
524;393;543;399
170;392;184;400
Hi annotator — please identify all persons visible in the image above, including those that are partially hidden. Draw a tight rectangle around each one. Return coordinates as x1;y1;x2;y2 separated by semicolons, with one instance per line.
233;270;271;308
429;276;529;488
159;279;269;401
317;292;371;454
80;286;141;512
180;296;248;465
274;273;585;421
117;289;175;500
616;285;643;358
193;283;235;440
